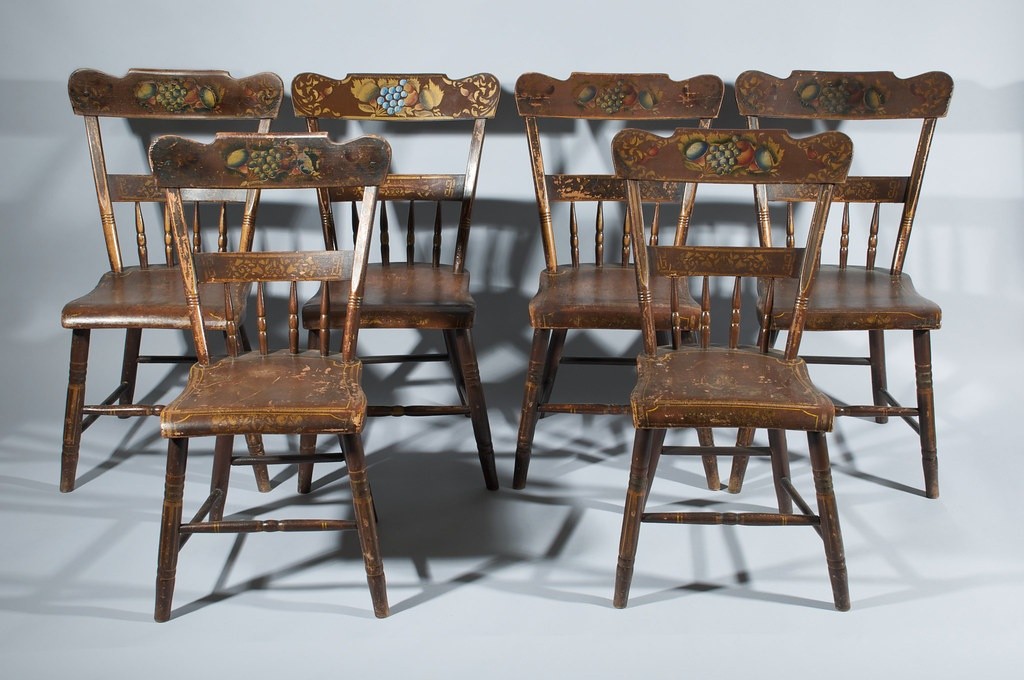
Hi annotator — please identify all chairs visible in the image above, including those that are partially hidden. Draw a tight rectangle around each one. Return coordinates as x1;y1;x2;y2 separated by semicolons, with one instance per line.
728;70;955;499
60;68;283;495
290;70;500;495
144;132;394;623
610;126;855;612
510;72;722;492
